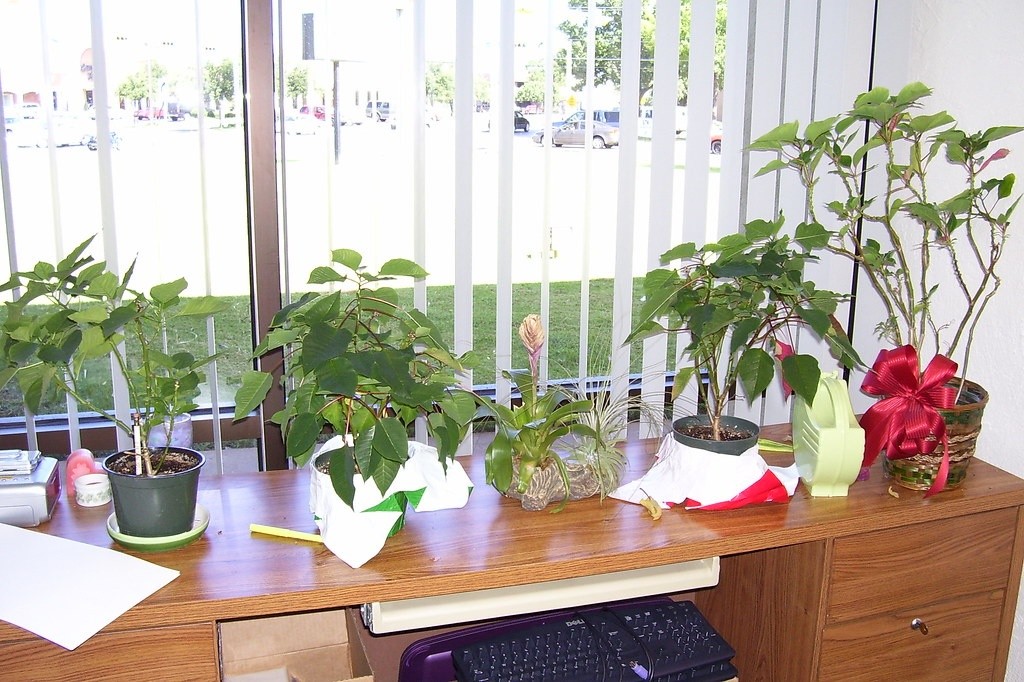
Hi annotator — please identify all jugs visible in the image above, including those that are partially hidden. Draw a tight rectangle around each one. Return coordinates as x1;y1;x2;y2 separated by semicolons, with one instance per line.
792;371;866;499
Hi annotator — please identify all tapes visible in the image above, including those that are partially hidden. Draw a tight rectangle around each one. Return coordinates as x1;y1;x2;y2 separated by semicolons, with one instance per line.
75;474;113;507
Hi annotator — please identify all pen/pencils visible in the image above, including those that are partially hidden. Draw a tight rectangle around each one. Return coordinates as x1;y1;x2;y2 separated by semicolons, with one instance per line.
250;524;324;543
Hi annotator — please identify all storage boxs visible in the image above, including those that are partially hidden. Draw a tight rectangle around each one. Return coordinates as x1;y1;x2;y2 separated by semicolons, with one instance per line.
218;609;377;682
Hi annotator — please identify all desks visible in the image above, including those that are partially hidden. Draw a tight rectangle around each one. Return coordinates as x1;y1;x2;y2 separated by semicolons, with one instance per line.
0;413;1024;682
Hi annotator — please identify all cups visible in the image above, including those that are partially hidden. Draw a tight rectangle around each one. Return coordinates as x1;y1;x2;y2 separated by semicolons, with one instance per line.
147;411;194;448
75;473;112;508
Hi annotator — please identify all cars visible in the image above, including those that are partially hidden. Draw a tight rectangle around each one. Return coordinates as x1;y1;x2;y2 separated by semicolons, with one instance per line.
364;100;390;123
555;108;723;155
531;119;619;149
4;102;190;150
275;103;360;137
487;111;530;133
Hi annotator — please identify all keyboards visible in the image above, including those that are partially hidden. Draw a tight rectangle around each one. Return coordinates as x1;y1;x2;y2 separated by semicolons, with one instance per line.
453;600;738;682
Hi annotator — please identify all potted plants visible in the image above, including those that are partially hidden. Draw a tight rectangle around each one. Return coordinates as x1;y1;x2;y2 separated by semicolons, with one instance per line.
618;210;885;505
0;230;233;552
478;311;696;513
743;78;1024;495
233;246;483;569
